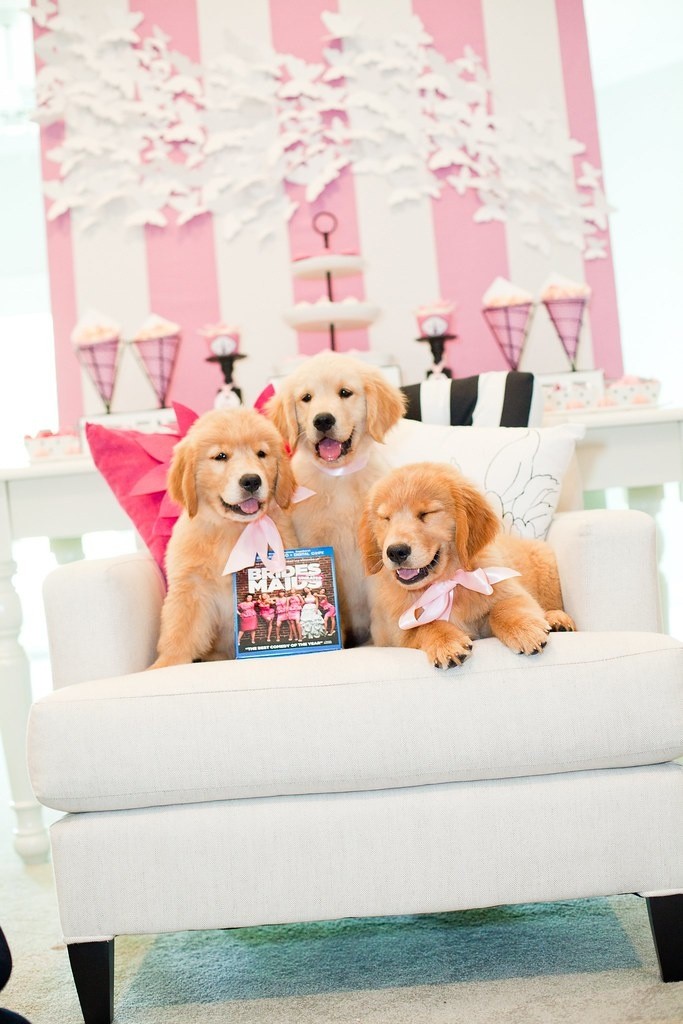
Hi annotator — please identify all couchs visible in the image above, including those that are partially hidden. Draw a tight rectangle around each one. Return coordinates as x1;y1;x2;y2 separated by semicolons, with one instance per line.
24;507;683;1024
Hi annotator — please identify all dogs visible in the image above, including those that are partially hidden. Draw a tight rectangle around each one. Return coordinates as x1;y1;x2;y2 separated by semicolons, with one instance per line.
265;355;409;650
142;404;301;672
356;460;579;671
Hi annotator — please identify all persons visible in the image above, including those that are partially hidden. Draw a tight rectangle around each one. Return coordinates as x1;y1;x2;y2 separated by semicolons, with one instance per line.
238;588;336;644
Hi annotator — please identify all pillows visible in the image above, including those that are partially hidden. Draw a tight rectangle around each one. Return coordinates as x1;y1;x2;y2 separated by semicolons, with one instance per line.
84;374;576;599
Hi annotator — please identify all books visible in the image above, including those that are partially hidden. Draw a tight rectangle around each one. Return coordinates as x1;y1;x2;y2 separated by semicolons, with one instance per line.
231;546;342;659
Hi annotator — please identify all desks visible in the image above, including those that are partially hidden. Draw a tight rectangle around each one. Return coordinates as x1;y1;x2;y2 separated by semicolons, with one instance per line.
0;399;683;865
